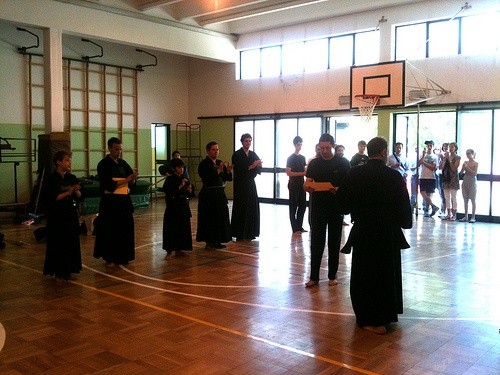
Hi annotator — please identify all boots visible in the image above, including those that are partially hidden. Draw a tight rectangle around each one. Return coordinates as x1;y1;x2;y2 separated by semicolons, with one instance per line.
442;208;451;220
450;209;457;221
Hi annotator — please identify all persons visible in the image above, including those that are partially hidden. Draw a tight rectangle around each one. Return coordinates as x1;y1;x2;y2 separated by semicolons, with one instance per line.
433;141;461;221
303;133;350;287
161;158;193;260
171;149;192;193
386;142;410;187
344;137;412;335
195;140;235;251
350;141;369;168
458;149;479;223
38;151;88;283
286;136;307;235
231;133;262;242
409;145;426;215
92;138;137;266
420;140;439;218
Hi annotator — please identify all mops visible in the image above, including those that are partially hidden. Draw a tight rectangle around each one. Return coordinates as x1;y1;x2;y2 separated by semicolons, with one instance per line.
21;168;44;226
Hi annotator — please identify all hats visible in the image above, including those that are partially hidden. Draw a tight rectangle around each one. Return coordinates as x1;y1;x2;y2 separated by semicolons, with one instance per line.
425;140;434;145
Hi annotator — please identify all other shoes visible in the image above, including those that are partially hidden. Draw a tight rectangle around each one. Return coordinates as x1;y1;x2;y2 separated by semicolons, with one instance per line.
343;221;349;226
430;205;439;216
329;279;337;286
306;280;315;286
470;218;476;223
214;243;226;249
296;231;302;235
423;207;430;217
459;218;468;221
364;325;385;335
300;229;308;232
55;273;71;280
166;253;172;259
205;243;213;250
175;252;190;257
